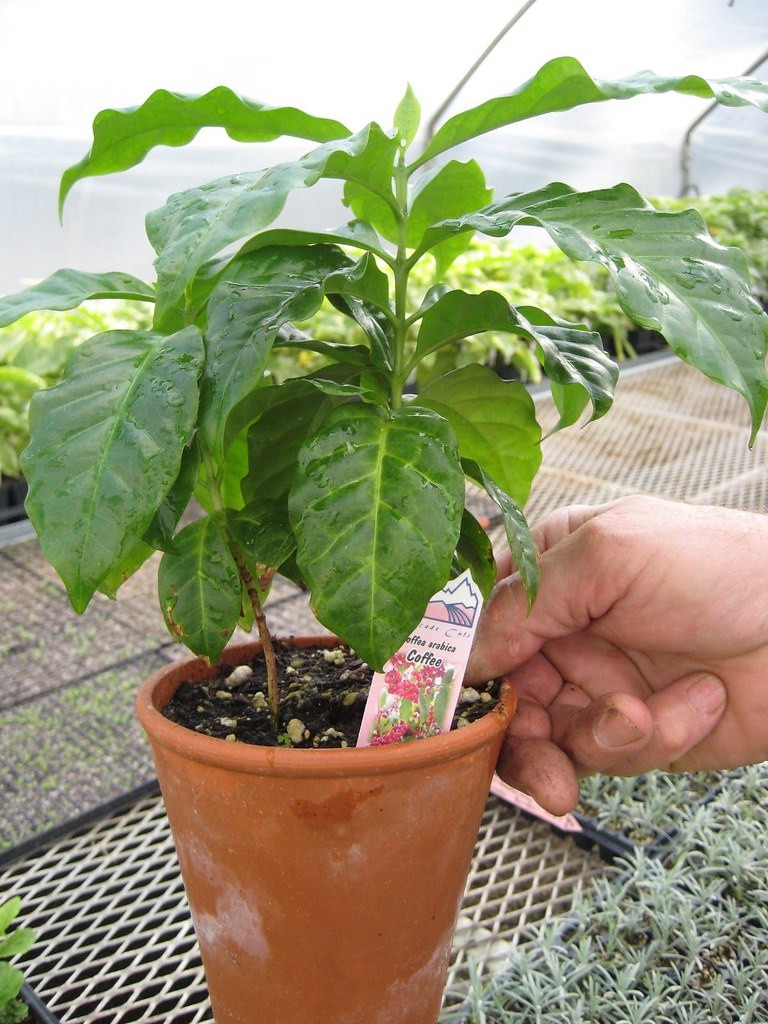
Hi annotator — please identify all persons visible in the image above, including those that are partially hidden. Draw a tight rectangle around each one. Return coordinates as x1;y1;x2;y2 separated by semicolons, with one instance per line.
462;495;766;816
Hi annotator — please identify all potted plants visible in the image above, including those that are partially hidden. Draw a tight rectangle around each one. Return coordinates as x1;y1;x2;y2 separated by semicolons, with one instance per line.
0;0;768;1024
